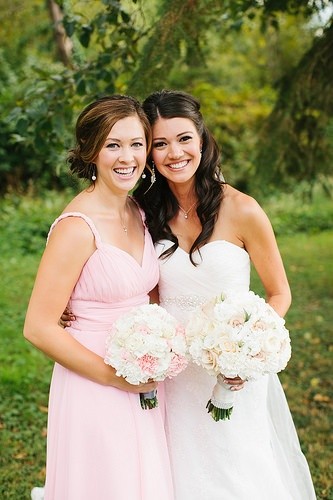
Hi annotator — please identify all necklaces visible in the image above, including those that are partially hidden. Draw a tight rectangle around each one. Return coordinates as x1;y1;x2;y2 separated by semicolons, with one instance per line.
91;185;129;238
176;198;200;219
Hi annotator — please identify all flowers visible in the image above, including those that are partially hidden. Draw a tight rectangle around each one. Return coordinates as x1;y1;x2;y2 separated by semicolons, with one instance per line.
185;289;293;422
104;303;191;411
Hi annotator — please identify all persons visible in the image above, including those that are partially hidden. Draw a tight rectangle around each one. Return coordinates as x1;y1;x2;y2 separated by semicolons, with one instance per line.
21;93;182;500
56;89;318;500
